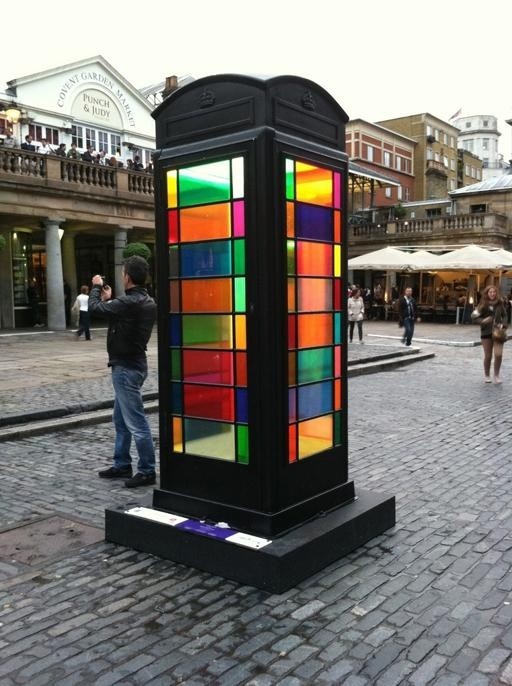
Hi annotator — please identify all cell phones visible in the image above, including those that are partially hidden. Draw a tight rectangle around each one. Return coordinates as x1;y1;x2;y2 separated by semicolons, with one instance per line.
101;276;106;289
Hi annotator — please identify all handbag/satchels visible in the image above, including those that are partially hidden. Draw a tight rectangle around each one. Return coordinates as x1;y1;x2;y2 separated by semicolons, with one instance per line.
491;325;508;343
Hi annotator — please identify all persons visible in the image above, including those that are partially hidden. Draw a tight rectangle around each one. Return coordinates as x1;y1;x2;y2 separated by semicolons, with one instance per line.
72;285;92;341
63;280;72;326
87;255;156;487
347;281;383;320
21;133;156;174
348;289;365;344
469;285;509;384
28;281;46;328
399;286;421;346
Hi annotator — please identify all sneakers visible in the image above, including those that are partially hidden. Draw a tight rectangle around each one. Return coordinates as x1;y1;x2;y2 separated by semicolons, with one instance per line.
125;470;156;488
98;465;133;478
483;375;502;385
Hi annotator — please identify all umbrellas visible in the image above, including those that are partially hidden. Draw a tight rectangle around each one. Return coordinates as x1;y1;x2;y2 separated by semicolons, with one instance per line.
348;247;510;302
